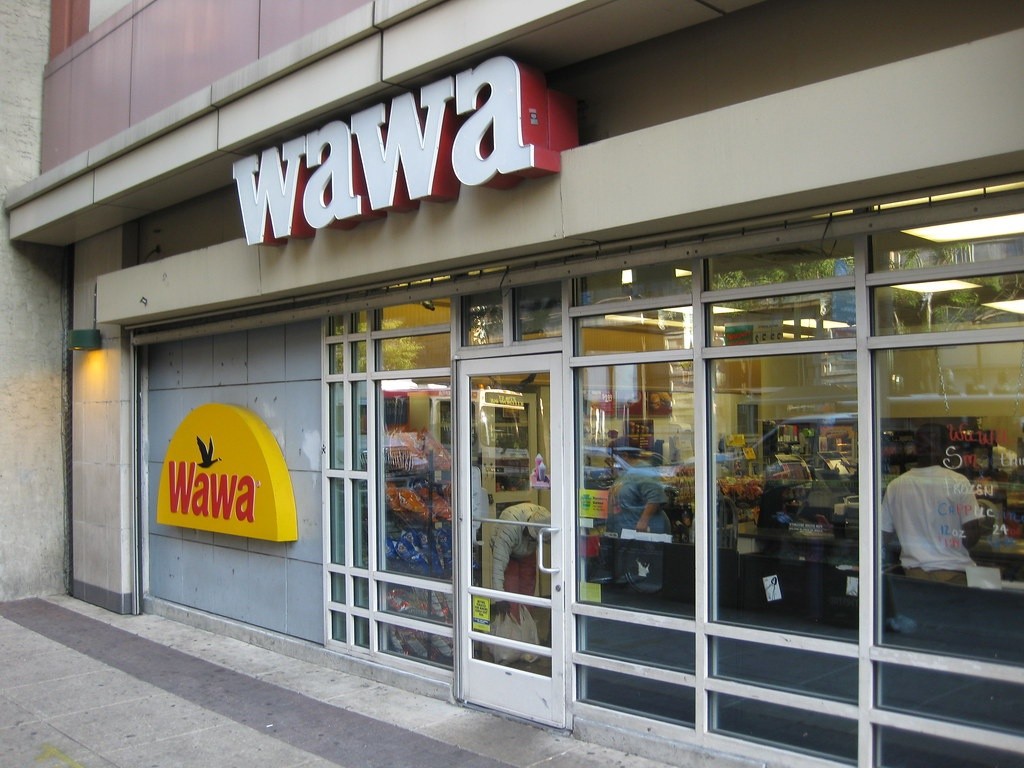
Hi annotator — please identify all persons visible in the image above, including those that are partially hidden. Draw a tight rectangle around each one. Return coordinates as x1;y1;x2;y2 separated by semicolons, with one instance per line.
489;503;551;623
602;424;1024;609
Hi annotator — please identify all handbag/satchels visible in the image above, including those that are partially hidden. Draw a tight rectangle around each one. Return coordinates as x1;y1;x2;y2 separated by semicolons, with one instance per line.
624;555;666;594
489;605;540;666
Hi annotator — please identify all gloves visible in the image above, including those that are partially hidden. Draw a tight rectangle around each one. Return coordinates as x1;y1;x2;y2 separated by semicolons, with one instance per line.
490;601;510;618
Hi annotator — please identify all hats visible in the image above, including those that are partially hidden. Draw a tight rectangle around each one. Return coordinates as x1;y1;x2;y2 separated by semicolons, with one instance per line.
528;509;551;542
